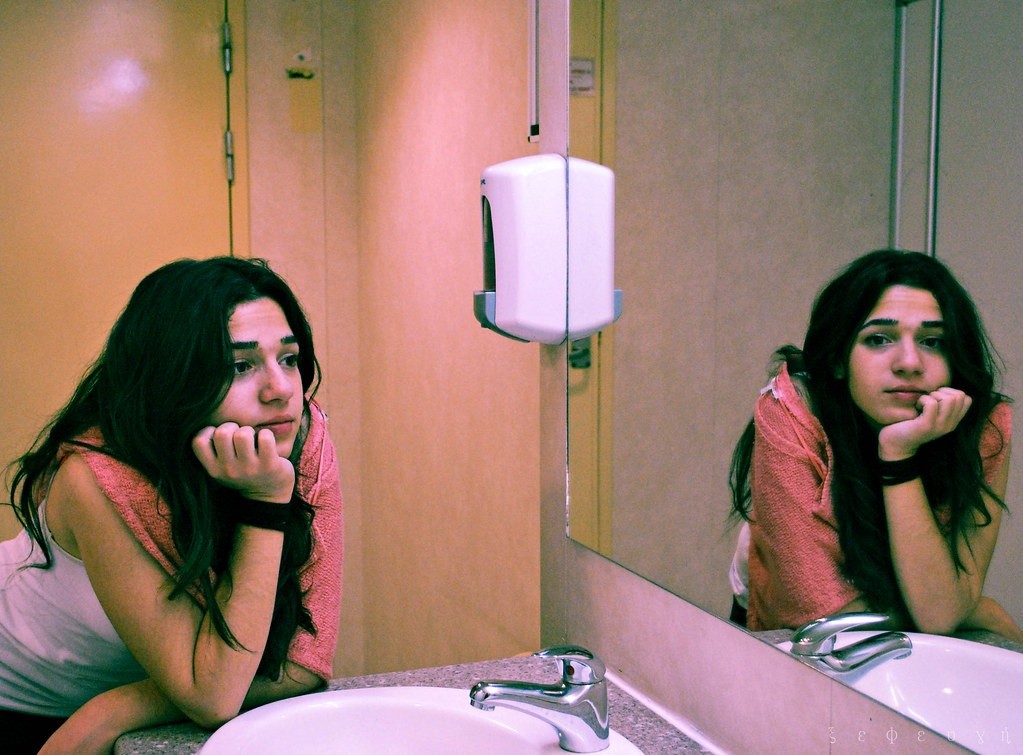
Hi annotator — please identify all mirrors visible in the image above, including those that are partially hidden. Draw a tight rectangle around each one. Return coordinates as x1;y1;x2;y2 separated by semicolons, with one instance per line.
562;0;1023;755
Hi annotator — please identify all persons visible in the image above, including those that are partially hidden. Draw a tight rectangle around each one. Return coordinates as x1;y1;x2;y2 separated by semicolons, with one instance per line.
719;247;1023;645
0;256;344;754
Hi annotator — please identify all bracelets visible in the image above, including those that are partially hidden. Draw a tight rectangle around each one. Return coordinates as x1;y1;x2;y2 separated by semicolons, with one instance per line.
233;495;290;533
878;454;921;487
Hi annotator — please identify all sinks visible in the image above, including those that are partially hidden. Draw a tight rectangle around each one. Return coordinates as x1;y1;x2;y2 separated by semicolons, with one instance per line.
198;687;644;754
772;630;1023;755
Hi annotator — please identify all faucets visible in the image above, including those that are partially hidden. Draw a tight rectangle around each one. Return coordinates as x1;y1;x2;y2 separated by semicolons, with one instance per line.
790;613;912;688
468;645;609;753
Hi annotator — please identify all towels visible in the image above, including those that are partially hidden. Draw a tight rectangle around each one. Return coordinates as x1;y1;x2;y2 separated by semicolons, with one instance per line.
745;359;1013;633
55;392;345;680
